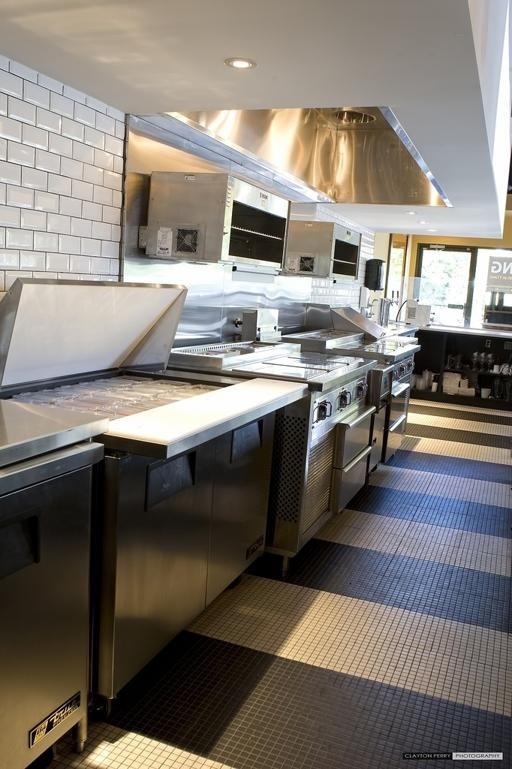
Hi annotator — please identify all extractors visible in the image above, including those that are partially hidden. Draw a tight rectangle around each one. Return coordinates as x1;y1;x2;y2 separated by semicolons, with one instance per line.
130;103;452;209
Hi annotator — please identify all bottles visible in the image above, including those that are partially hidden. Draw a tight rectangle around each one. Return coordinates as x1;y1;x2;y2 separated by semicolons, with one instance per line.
487;353;493;373
471;352;478;372
479;353;486;373
431;383;438;392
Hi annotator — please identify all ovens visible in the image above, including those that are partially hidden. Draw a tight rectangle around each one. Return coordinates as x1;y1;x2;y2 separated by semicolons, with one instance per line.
284;219;362;280
292;355;415;553
147;172;290;277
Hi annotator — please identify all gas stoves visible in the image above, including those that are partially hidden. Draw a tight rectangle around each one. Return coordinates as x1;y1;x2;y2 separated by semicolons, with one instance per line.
167;328;422;386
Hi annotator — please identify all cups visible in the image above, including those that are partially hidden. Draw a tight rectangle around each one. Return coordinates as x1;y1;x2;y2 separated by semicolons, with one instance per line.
481;388;491;399
494;363;512;376
